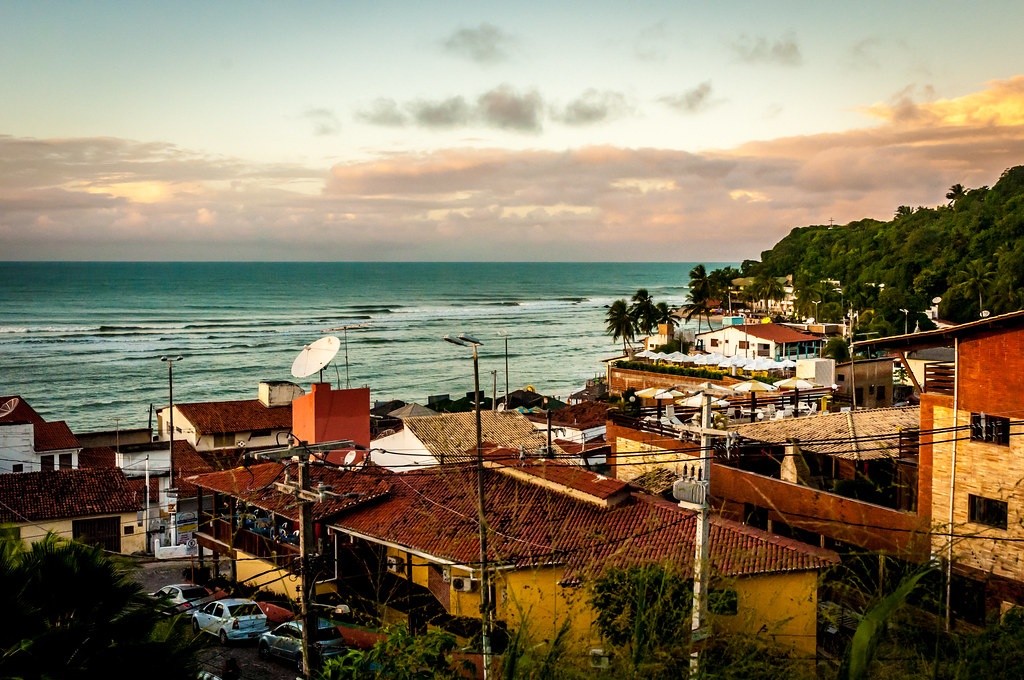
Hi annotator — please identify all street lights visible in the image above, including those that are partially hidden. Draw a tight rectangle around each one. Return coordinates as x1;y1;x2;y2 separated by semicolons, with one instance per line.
898;309;909;357
160;355;185;546
443;331;496;680
812;300;823;324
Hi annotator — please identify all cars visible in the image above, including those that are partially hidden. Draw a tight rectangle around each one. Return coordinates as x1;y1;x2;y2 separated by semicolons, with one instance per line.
256;618;349;675
146;583;216;619
191;597;269;644
